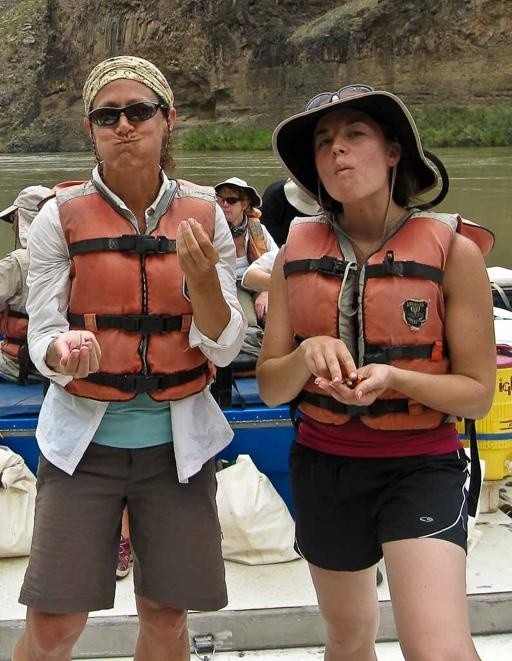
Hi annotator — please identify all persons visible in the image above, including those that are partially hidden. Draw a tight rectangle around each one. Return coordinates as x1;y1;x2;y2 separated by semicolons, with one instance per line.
13;56;247;661
255;83;497;660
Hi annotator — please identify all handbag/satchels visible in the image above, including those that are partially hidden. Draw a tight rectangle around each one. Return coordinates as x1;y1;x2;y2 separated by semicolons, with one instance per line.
214;452;303;566
0;446;37;560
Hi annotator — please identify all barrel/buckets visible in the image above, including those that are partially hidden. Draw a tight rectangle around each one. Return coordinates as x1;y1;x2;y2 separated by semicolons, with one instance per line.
453;345;512;480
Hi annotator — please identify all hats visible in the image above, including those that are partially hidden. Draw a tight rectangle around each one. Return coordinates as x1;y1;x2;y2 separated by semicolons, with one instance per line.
0;184;52;251
271;86;449;215
212;177;266;212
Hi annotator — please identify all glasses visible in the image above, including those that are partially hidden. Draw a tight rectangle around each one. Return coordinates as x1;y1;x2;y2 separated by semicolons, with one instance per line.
89;103;168;128
306;84;374;110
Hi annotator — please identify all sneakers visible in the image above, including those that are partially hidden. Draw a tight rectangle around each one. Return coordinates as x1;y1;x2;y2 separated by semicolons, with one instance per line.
116;536;133;580
